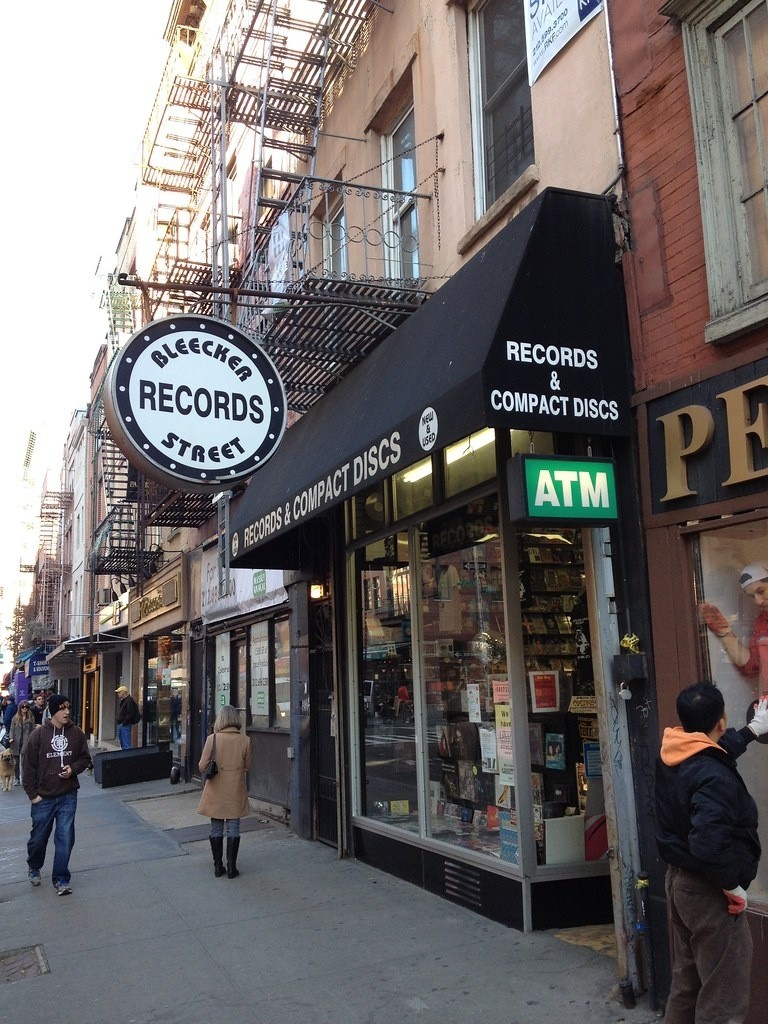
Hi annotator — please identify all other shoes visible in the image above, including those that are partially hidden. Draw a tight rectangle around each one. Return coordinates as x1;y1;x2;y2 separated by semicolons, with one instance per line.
175;738;183;744
14;779;20;786
21;779;24;785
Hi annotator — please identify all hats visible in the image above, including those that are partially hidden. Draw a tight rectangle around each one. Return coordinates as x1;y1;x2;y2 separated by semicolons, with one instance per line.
114;686;128;692
6;697;15;702
739;563;768;589
49;695;70;717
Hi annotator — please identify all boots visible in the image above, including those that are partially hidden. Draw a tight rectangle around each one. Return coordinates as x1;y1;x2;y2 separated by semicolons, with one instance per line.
227;836;240;879
209;835;226;877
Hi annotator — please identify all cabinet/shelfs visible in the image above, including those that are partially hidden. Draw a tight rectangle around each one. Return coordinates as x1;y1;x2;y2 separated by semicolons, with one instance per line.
407;528;602;861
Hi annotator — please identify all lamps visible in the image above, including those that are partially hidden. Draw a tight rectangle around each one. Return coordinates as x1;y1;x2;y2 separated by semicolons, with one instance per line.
402;427;496;483
110;541;181;602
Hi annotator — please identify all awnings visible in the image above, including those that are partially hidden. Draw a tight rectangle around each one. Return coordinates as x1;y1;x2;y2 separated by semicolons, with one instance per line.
225;187;633;568
46;633;129;662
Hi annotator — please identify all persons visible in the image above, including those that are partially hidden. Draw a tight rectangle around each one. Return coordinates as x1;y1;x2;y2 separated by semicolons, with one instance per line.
0;691;90;897
148;689;182;742
114;686;138;750
654;560;768;1024
196;705;251;879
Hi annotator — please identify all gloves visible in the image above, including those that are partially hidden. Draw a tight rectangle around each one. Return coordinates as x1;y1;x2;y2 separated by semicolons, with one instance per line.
722;885;748;914
700;604;730;637
748;694;768;738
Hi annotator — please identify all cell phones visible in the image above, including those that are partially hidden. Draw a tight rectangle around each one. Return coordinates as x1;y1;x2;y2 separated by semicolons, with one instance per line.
61;771;68;775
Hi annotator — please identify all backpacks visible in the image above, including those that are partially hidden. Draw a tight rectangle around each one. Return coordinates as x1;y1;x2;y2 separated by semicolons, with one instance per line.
126;697;140;725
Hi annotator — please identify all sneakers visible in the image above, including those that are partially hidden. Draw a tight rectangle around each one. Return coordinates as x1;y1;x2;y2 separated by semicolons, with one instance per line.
53;878;72;896
28;866;41;885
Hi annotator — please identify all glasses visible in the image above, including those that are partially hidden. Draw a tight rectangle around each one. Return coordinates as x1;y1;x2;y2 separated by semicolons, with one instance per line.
58;703;72;710
20;704;29;709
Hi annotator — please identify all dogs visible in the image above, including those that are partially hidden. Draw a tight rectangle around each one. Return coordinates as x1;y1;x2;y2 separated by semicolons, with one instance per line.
0;748;17;792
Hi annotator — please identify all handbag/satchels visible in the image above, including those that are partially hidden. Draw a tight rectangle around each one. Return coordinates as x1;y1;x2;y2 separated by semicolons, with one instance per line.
204;733;218;780
0;730;11;748
